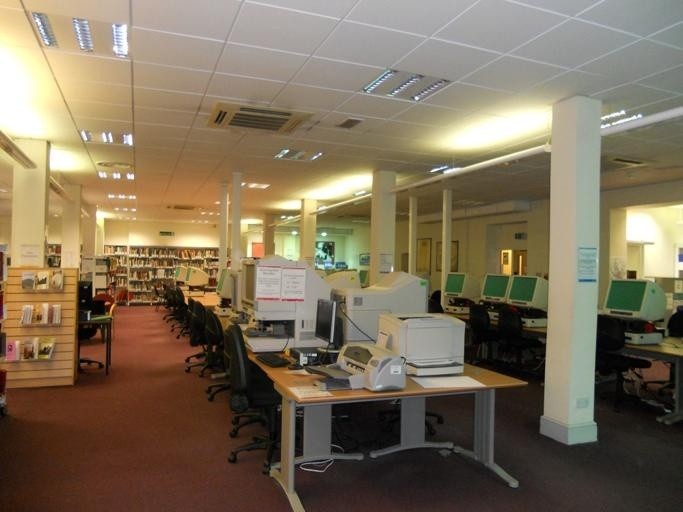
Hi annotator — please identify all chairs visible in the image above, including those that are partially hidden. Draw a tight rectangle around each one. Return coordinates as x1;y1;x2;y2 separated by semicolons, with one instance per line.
94;293;116;343
78;328;104;372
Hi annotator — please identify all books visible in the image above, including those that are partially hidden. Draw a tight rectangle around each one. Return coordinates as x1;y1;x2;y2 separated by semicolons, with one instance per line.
3;269;62;361
104;245;232;307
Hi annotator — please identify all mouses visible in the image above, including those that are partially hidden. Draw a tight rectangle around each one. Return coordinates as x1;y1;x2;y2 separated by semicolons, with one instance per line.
288;363;302;369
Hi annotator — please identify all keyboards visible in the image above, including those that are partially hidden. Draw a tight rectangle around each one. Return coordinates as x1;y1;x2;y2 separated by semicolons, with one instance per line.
256;353;291;366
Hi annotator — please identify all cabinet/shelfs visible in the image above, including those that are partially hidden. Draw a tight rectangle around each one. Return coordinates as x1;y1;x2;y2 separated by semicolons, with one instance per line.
104;244;219;307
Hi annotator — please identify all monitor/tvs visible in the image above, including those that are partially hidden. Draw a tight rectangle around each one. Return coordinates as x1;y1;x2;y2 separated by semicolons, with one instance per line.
602;281;668;334
241;264;256;301
79;281;92;309
325;272;430;345
174;267;187;286
480;272;510;310
322;270;362;294
315;297;344;353
186;267;210;289
508;275;549;317
214;268;236;308
445;272;479;304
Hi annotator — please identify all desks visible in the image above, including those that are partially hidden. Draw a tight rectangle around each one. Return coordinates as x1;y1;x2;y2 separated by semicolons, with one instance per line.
154;284;683;512
78;315;112;376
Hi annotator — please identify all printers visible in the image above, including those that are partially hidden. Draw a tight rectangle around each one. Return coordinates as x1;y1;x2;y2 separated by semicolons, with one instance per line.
305;344;406;392
376;314;465;376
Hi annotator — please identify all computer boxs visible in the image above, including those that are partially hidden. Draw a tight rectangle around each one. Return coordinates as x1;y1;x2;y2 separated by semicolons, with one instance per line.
291;345;338;366
79;311;92;321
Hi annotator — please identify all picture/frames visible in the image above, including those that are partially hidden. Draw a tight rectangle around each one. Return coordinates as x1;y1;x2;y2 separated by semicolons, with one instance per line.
416;238;432;276
436;240;459;272
